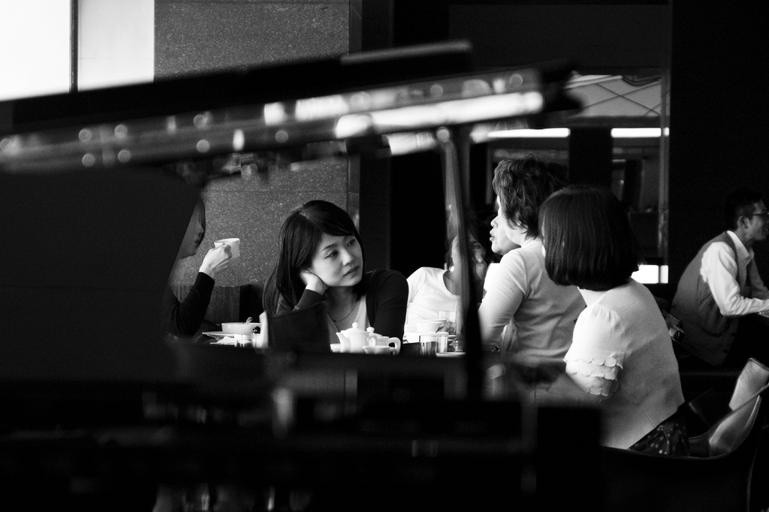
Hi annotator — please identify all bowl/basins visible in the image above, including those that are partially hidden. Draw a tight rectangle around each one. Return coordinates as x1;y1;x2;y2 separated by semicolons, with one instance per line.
221;322;260;334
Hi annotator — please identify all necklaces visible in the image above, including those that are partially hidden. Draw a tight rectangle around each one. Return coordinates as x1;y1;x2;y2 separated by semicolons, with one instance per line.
331;298;358;323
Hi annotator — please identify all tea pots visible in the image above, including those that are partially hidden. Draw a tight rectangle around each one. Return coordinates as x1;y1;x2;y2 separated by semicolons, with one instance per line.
329;322;402;355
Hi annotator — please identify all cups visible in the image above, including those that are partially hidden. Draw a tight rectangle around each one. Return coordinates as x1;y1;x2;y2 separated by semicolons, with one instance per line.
417;320;464;356
213;238;243;260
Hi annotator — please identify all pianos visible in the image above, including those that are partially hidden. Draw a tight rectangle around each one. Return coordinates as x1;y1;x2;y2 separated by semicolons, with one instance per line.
1;40;609;511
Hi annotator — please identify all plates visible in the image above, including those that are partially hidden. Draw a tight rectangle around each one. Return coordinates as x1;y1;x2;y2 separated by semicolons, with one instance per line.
201;331;249;340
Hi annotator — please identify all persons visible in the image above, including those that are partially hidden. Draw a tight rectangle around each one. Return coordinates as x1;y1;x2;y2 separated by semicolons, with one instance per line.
667;188;769;370
401;211;498;351
538;185;692;510
261;198;409;356
159;198;234;335
476;154;586;386
482;193;519;298
